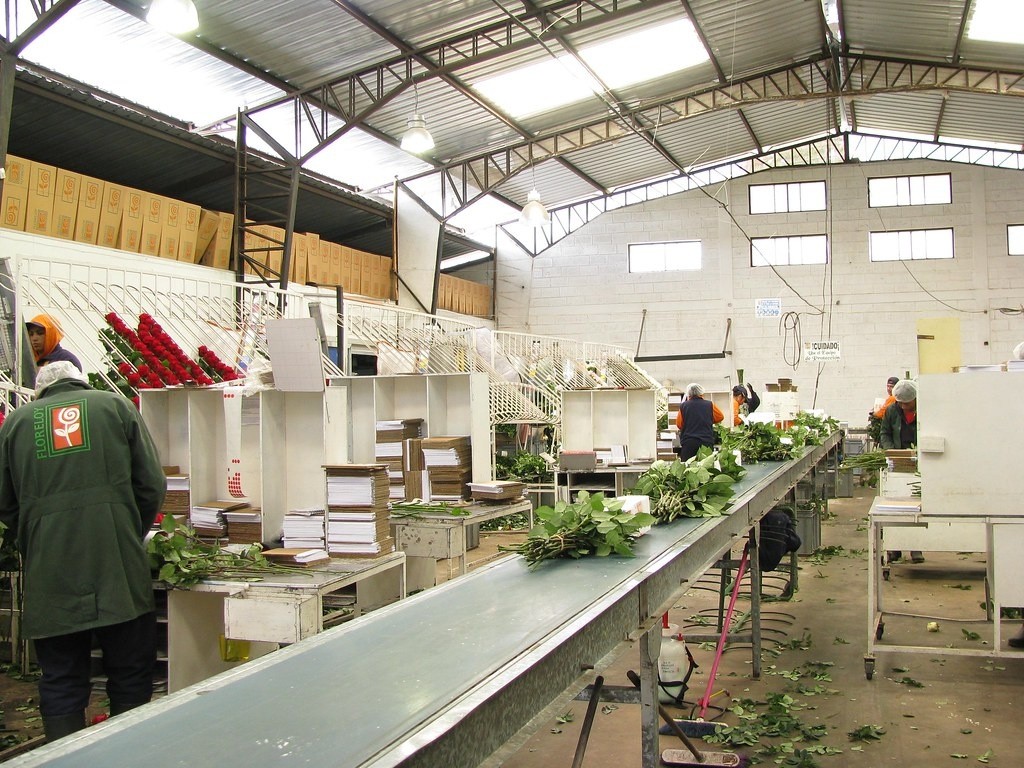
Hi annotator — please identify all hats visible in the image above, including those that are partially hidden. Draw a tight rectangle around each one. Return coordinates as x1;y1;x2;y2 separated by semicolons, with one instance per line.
737;386;747;399
25;321;45;331
37;348;83;372
887;377;898;385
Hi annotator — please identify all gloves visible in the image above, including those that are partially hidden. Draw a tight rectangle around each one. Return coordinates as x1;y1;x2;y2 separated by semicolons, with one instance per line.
747;383;754;393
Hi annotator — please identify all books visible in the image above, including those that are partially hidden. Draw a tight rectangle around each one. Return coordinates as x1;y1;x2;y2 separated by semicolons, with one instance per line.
876;448;918;514
158;418;527;568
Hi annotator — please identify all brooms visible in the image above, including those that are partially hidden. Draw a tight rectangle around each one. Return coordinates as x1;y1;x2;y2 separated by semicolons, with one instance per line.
626;670;751;768
658;550;752;738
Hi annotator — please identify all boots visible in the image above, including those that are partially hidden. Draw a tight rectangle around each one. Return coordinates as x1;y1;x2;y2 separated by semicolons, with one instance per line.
109;698;149;718
42;716;85;743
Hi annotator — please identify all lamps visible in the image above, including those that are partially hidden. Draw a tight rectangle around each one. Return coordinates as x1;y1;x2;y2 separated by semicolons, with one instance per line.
518;165;550;227
401;85;435;153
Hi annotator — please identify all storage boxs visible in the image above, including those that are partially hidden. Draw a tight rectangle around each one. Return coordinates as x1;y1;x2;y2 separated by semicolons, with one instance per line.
24;161;56;236
96;181;125;249
193;207;222;264
116;187;146;253
198;211;234;270
176;202;201;264
138;192;166;257
288;232;393;300
158;197;183;260
0;154;31;231
73;175;105;245
244;218;285;287
51;168;81;240
437;273;490;318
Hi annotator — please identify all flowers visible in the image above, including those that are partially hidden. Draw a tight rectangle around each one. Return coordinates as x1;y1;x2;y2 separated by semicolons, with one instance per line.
87;313;238;413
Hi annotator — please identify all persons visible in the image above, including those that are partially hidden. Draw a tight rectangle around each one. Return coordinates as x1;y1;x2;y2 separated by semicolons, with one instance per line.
25;312;83;374
880;380;925;563
874;377;899;418
732;383;761;425
676;383;724;462
0;361;167;742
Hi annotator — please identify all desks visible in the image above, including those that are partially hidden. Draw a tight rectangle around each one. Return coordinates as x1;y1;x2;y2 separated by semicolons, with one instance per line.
0;461;674;696
865;466;1024;680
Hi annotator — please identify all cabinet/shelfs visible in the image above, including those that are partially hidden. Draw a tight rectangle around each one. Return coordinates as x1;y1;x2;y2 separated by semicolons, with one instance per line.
90;582;278;696
0;571;22;664
